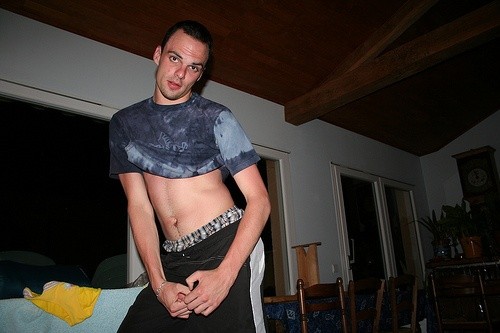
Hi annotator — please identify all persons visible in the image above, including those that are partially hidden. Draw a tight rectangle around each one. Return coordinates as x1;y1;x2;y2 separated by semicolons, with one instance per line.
109;20;271;333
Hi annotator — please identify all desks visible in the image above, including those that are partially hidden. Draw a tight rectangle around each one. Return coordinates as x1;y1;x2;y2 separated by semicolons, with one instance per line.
264;293;375;333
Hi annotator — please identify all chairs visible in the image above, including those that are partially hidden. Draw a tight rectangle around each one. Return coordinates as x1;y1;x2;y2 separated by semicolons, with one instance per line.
296;274;419;333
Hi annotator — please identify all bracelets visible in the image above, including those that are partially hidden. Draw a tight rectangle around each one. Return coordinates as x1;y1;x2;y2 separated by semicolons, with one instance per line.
156;281;166;297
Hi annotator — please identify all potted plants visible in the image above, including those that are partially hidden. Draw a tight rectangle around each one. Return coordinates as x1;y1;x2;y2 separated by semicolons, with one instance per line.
442;200;481;259
409;208;452;259
